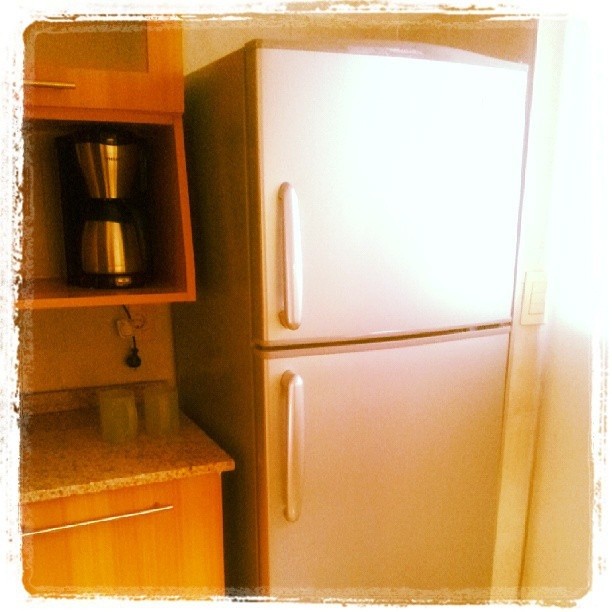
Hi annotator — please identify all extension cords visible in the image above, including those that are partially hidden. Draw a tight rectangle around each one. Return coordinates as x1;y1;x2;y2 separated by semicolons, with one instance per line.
55;131;155;286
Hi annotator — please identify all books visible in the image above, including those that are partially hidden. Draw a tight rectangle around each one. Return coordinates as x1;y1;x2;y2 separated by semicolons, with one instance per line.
19;14;189;113
12;114;197;311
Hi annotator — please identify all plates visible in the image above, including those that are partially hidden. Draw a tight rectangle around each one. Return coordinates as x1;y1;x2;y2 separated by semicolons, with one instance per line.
18;473;227;602
